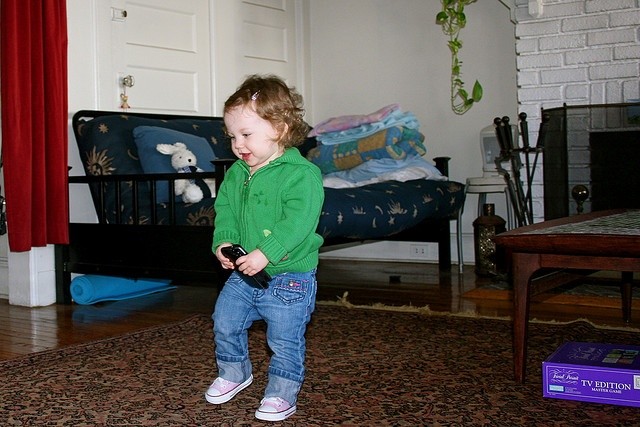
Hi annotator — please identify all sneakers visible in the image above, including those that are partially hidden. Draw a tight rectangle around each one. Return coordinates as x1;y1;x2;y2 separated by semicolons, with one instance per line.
204;373;254;405
254;396;297;422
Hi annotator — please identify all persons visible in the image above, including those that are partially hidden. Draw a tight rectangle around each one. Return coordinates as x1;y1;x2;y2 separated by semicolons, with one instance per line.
204;75;325;422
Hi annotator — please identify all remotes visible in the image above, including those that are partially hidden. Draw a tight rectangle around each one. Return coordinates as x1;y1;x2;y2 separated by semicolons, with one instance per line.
236;246;273;283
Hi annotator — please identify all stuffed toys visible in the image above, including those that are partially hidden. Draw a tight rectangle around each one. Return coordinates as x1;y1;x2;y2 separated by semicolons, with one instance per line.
156;143;216;203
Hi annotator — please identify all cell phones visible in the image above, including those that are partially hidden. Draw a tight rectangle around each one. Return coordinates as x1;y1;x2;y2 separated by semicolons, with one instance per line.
221;248;270;290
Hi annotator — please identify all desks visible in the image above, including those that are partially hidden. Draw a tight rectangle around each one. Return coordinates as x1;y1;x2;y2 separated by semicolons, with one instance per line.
491;207;640;387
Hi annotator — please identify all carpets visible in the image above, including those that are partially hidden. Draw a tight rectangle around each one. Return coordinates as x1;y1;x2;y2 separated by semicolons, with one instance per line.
1;301;640;427
460;269;640;311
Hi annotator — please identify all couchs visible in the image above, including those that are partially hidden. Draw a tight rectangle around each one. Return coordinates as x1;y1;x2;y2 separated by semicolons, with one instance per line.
73;111;467;275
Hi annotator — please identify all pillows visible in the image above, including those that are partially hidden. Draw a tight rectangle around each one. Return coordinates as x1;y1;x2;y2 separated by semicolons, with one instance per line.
133;126;218;203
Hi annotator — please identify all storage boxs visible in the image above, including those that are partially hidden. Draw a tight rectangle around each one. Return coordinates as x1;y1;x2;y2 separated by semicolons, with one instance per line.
542;342;640;407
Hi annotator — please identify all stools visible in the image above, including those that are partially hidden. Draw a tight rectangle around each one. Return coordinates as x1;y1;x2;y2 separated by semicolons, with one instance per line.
457;177;523;282
